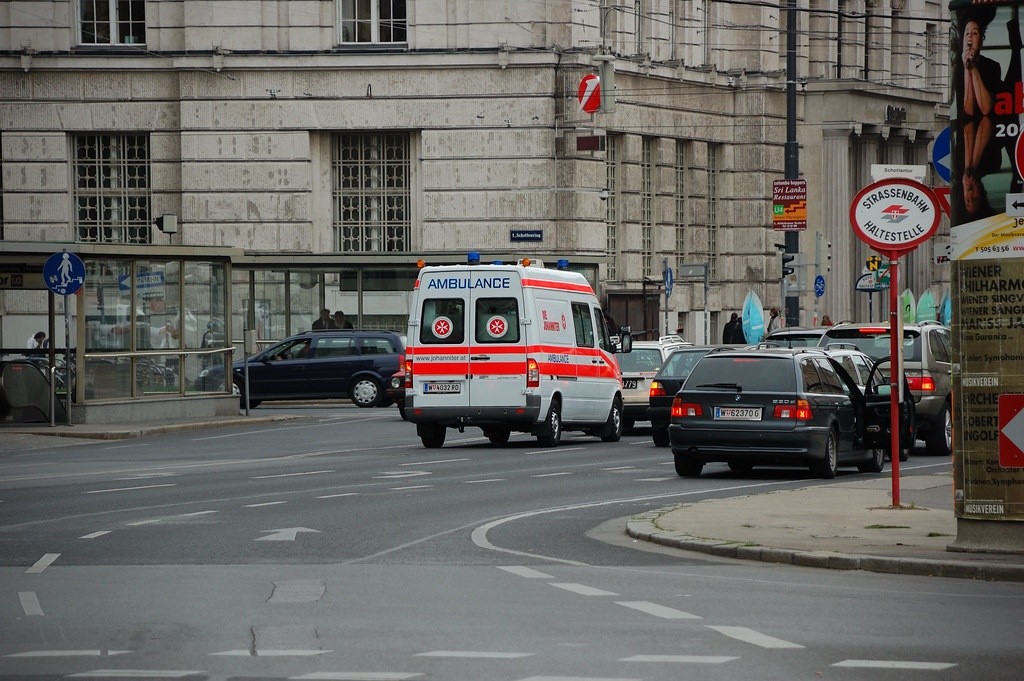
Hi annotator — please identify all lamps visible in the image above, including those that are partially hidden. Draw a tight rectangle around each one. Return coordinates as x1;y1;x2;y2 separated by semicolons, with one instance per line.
152;214;178;244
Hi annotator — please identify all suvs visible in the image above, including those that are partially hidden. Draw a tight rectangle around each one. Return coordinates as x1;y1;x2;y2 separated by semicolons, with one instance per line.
644;344;749;448
192;327;408;410
596;333;695;434
813;320;958;457
666;347;918;478
755;341;912;460
760;325;829;350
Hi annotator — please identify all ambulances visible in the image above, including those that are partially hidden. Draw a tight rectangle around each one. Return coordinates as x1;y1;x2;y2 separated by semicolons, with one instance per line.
400;251;634;449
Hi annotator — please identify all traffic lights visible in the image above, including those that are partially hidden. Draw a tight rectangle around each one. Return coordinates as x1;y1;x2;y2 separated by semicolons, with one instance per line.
775;251;795;279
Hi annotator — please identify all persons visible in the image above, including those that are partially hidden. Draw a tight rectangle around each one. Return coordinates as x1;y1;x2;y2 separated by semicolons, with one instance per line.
960;118;1001;212
312;310;354;331
722;307;783;344
27;332;46;360
275;341;310;361
957;21;1001;113
198;317;223;373
819;316;831;326
161;320;192;388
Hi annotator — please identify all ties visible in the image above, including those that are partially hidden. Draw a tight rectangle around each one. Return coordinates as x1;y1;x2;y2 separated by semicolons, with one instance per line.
324;321;326;329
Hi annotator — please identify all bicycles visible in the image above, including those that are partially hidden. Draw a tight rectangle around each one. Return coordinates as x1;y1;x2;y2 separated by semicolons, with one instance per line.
0;353;174;402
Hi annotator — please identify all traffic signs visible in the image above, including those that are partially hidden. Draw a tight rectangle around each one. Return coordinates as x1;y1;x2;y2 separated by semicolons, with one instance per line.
119;270;164;291
